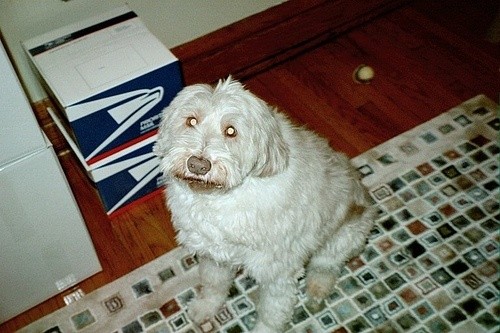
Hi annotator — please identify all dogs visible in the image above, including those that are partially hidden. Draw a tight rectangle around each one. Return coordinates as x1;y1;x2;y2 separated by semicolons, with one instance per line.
151;75;377;333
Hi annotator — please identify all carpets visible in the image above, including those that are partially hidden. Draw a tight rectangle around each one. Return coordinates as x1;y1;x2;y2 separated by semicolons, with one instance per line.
13;93;500;332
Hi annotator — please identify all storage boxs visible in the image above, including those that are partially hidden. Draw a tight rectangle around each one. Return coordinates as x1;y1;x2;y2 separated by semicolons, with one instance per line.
1;130;104;326
17;3;186;166
0;42;49;170
45;106;170;221
